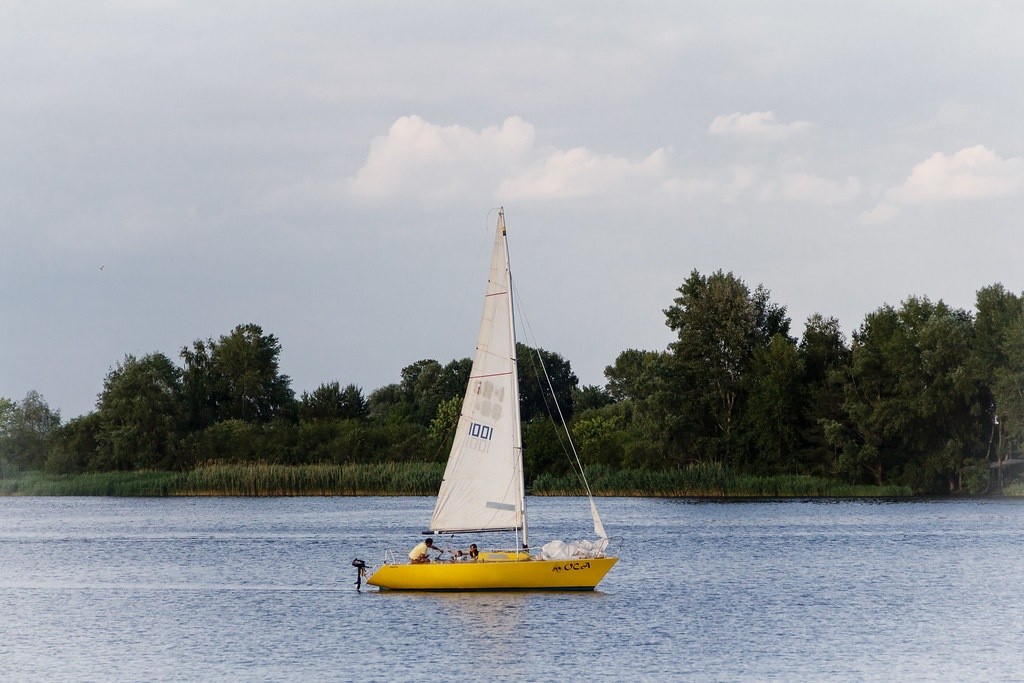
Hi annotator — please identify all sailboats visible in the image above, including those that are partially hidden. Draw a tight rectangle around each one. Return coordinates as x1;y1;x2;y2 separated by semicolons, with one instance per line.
351;208;621;594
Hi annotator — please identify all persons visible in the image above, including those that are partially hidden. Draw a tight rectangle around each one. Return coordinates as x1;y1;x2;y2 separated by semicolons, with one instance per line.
470;544;479;561
408;538;443;564
453;550;464;561
